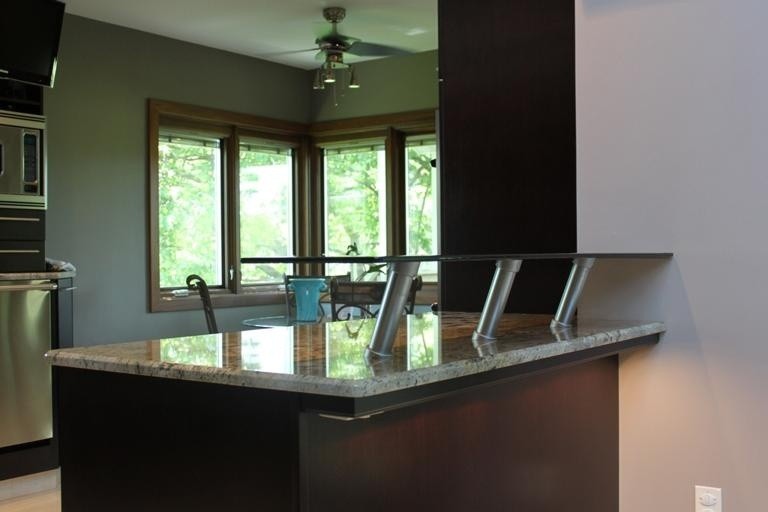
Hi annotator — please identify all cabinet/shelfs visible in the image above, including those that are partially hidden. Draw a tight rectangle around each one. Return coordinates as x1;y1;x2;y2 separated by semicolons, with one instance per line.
0;202;45;273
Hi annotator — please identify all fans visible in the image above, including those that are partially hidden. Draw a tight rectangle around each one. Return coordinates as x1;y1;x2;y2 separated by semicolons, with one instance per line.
257;5;424;108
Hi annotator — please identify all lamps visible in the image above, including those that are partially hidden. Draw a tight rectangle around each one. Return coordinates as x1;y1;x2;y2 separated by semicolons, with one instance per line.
312;62;361;108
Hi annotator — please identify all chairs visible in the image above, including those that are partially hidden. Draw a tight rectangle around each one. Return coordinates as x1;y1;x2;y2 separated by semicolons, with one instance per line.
332;276;421;321
284;272;351;322
187;274;219;334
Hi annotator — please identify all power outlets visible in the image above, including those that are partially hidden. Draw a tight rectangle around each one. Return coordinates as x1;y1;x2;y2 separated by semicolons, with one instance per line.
695;485;722;512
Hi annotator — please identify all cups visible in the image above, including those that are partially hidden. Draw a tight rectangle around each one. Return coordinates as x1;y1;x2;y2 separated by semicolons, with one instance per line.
350;257;364;282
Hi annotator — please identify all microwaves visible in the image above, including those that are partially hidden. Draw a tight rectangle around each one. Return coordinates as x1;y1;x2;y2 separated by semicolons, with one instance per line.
0;124;40;195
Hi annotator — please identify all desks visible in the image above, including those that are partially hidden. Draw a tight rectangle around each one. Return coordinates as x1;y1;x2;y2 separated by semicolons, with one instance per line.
45;252;672;512
241;304;433;328
